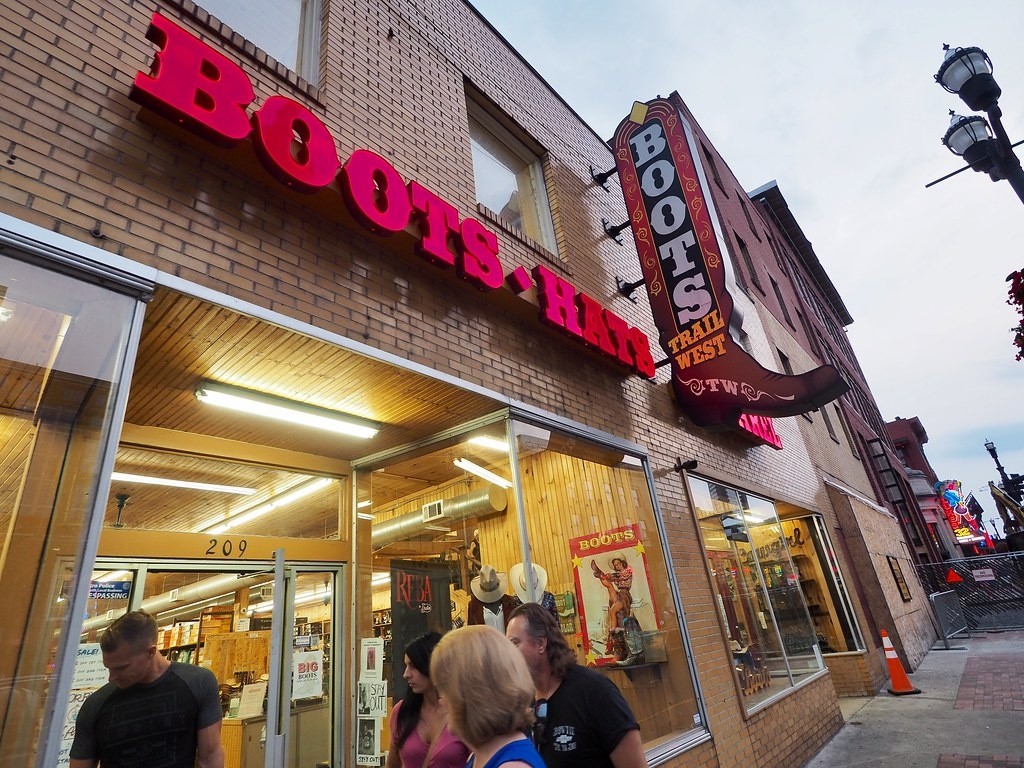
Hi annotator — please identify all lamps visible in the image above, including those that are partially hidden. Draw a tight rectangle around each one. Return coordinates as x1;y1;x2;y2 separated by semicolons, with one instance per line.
194;383;381;440
451;456;513;491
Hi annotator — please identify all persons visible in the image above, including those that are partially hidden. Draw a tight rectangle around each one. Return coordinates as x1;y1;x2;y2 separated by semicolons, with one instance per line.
386;602;648;768
69;608;225;768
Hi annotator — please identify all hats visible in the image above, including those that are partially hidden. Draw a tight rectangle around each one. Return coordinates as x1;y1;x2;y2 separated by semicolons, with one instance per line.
470;564;507;604
508;561;548;605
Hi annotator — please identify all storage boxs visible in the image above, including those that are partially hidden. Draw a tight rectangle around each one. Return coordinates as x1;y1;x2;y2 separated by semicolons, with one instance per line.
154;605;234;665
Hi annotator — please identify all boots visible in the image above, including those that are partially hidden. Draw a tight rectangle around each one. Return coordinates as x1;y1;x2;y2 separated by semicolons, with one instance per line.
615;618;646;666
604;626;627;667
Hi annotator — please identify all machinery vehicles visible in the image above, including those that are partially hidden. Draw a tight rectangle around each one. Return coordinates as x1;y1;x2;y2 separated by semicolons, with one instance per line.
988;479;1024;533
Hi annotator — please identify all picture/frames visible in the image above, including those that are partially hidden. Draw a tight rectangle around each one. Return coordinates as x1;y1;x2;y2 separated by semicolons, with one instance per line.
886;554;914;602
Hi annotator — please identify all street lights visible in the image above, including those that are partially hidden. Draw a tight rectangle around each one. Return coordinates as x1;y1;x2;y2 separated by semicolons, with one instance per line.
983;438;1022;527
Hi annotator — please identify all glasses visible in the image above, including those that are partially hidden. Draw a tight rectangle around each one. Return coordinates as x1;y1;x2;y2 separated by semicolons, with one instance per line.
531;697;549;746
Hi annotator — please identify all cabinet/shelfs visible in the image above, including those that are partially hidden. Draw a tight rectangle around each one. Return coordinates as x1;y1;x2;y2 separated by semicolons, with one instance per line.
157;609;233;666
293;608;395;664
222;703;331;768
741;554;829;626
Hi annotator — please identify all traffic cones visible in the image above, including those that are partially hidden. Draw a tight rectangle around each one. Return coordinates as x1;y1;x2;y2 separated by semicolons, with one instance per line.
879;628;923;695
